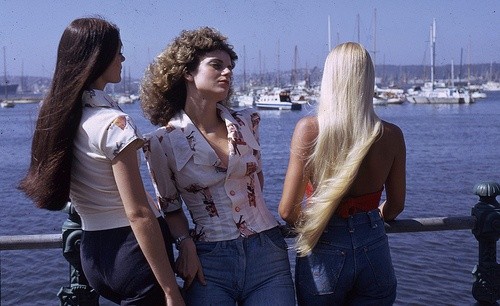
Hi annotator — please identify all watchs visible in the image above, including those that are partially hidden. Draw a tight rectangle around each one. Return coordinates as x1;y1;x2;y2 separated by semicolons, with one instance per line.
175;233;192;250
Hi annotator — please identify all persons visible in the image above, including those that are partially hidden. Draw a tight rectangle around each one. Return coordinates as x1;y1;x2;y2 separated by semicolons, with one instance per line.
278;40;407;306
137;26;297;306
17;17;185;306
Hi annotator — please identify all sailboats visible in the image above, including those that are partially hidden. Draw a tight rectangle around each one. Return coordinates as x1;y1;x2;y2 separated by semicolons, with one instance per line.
3;9;485;106
255;38;305;109
3;67;41;102
482;58;500;91
406;12;475;103
